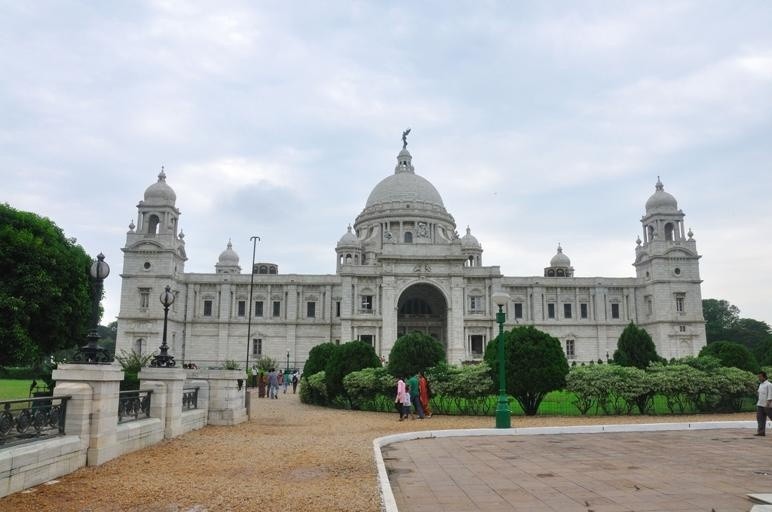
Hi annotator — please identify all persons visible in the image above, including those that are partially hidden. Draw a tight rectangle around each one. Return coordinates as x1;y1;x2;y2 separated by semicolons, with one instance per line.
258;367;303;399
416;369;433;418
405;371;425;419
752;371;772;436
394;375;406;420
251;365;258;388
381;356;386;367
403;385;416;420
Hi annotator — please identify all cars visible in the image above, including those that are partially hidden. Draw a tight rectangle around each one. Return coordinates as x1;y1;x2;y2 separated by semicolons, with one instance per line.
283;368;300;382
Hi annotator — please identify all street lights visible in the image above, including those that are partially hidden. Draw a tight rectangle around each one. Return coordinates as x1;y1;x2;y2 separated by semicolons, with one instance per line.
491;291;511;428
605;348;610;361
286;348;291;372
148;285;181;368
65;251;122;365
245;234;264;390
